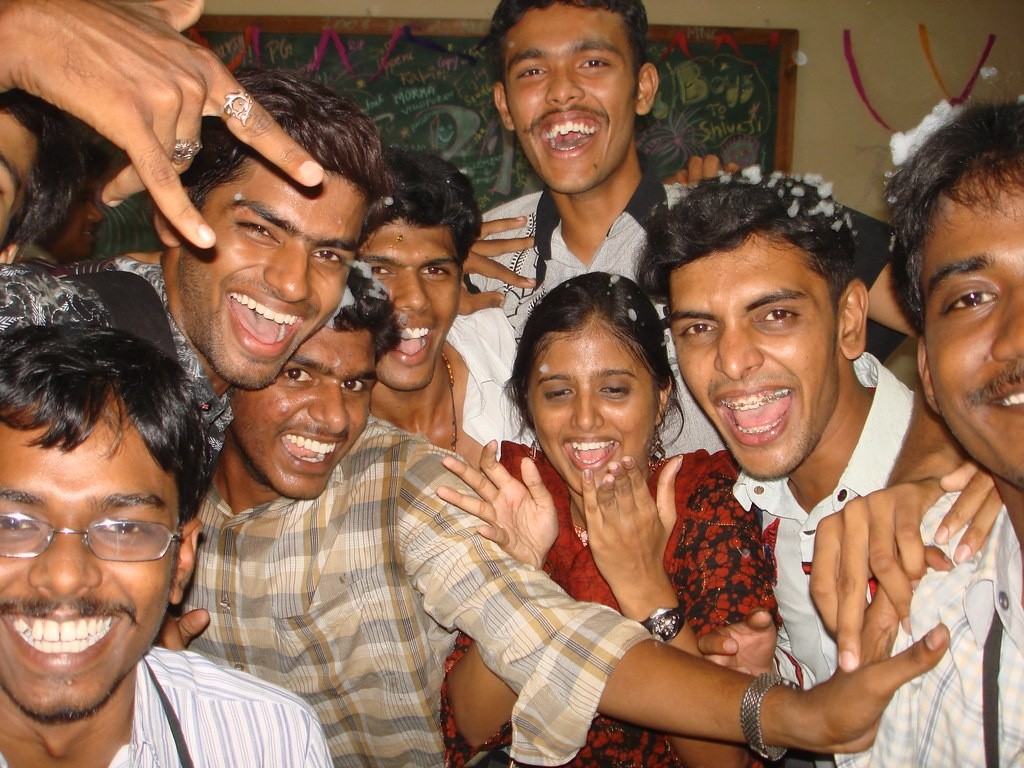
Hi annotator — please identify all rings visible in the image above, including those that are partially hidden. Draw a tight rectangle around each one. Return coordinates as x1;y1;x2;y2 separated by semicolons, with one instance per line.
174;133;203;165
221;85;255;126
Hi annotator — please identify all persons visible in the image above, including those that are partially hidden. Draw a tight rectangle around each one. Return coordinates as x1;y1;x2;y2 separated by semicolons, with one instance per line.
0;0;1024;768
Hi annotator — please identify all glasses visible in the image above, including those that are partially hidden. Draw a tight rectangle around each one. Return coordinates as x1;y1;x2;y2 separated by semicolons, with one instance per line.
0;513;186;562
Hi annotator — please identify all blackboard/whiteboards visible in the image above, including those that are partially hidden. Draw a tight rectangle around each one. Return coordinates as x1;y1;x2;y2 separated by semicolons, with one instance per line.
83;16;796;259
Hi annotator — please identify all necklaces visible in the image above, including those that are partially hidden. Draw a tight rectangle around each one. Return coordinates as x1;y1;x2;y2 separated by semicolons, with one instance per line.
440;351;457;453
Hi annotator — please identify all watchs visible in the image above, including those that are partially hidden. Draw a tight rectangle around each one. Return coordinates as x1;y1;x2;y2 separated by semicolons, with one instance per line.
637;604;684;644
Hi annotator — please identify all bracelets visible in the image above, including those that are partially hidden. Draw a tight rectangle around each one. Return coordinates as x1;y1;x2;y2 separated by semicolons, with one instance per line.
739;666;803;763
